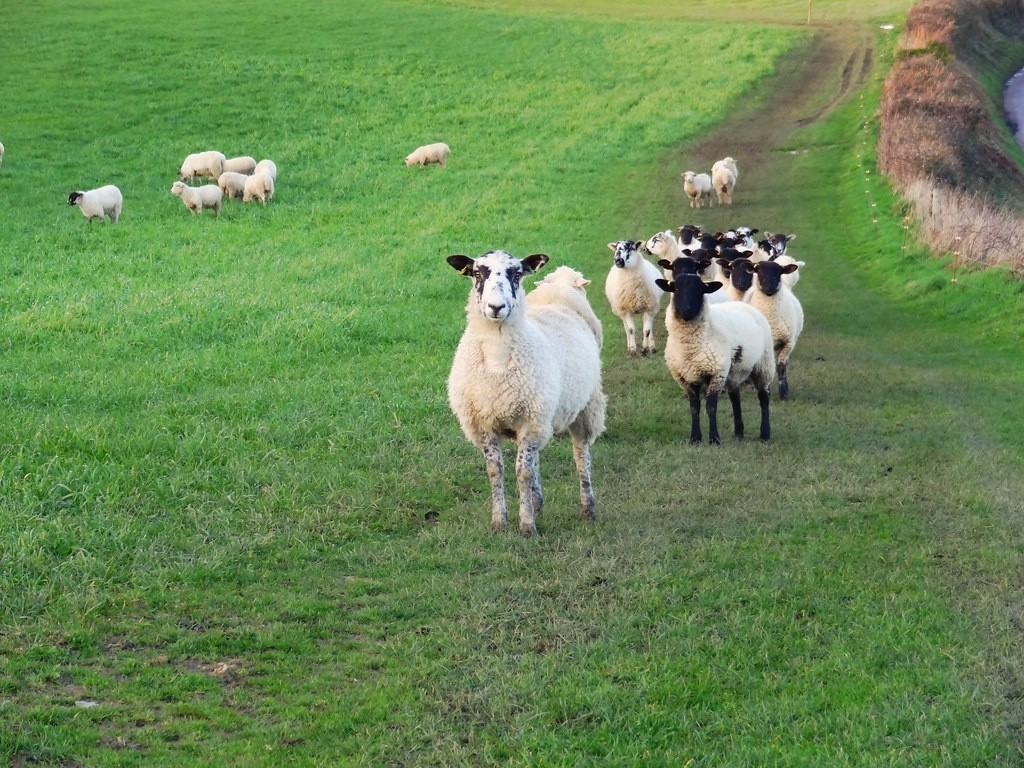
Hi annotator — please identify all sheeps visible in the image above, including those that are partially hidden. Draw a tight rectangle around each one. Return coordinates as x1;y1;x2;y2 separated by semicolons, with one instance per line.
66;184;123;225
404;142;451;168
170;150;277;218
606;223;806;445
680;157;739;209
446;250;605;541
0;142;5;165
